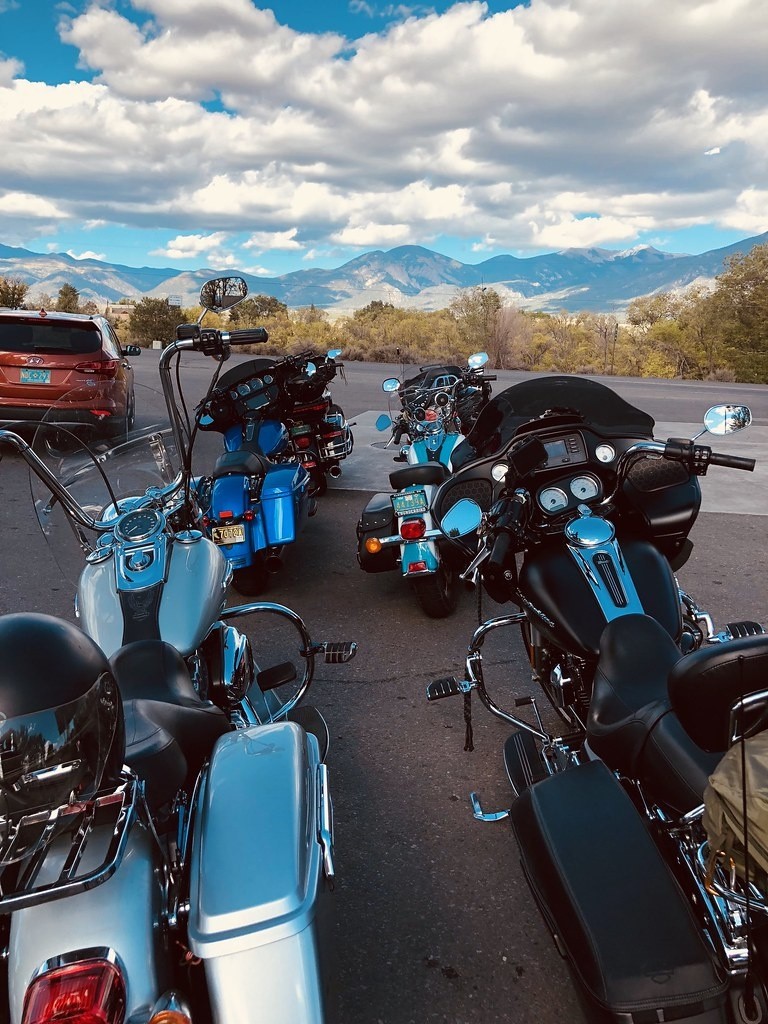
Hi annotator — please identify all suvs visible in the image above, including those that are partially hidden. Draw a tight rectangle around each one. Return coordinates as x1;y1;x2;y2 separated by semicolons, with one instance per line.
0;304;142;450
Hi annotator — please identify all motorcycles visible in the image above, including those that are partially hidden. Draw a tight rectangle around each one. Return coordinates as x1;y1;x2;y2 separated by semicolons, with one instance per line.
355;352;502;620
0;274;359;1024
179;346;348;596
386;374;768;1024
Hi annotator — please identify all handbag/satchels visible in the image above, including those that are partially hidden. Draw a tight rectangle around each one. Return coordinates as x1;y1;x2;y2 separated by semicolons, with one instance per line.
702;728;768;885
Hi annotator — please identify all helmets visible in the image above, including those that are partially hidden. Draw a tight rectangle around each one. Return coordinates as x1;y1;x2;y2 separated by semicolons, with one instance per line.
0;612;125;867
